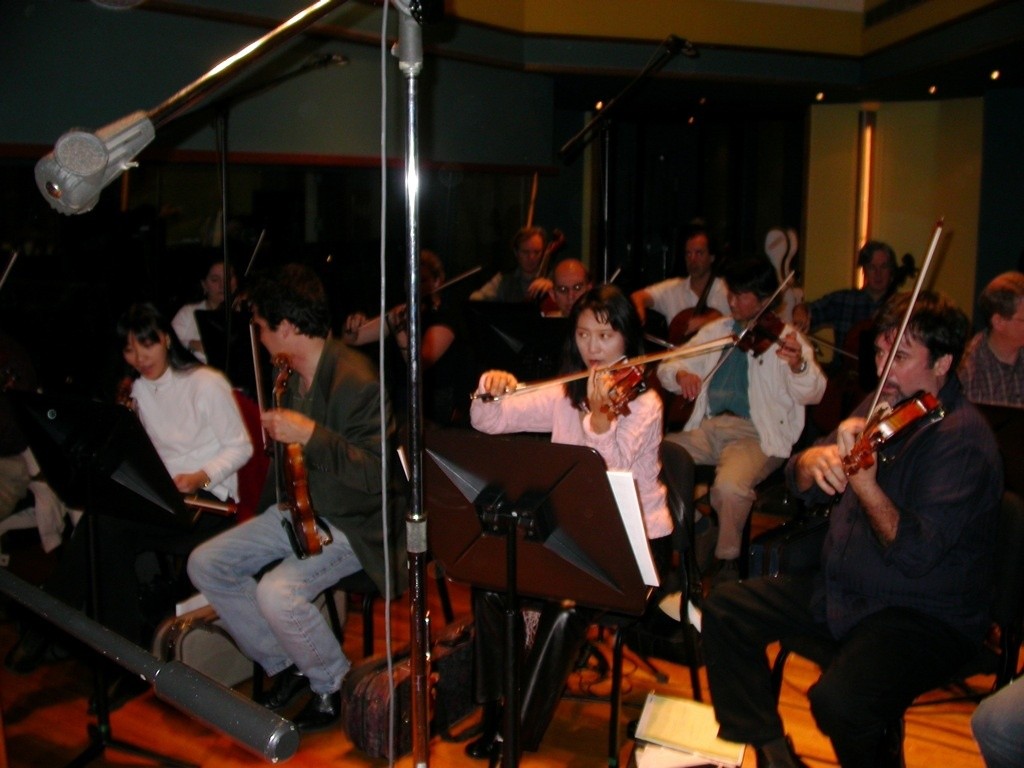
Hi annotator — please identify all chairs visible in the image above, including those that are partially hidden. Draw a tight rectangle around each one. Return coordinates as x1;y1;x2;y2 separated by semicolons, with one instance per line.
231;395;1024;768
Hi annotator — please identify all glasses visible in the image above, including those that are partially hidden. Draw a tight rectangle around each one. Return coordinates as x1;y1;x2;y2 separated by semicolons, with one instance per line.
559;280;587;293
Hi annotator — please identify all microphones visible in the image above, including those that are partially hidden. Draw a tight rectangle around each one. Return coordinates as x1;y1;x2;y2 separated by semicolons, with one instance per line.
667;36;697;55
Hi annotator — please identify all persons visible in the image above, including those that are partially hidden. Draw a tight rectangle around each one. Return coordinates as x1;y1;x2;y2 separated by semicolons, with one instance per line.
0;227;1024;768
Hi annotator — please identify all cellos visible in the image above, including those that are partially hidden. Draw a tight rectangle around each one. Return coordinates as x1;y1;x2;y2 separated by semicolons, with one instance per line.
813;252;920;435
645;240;739;423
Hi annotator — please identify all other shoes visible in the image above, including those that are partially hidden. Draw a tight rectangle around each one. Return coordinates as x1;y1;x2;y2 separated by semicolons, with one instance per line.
294;689;342;733
88;679;154;714
263;665;309;714
465;725;498;759
709;561;738;602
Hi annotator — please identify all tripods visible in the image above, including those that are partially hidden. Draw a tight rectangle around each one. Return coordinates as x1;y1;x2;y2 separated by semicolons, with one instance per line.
11;388;203;768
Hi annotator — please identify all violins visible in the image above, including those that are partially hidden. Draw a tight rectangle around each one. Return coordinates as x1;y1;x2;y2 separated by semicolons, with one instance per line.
269;352;333;561
736;309;807;375
841;389;946;478
577;354;662;423
